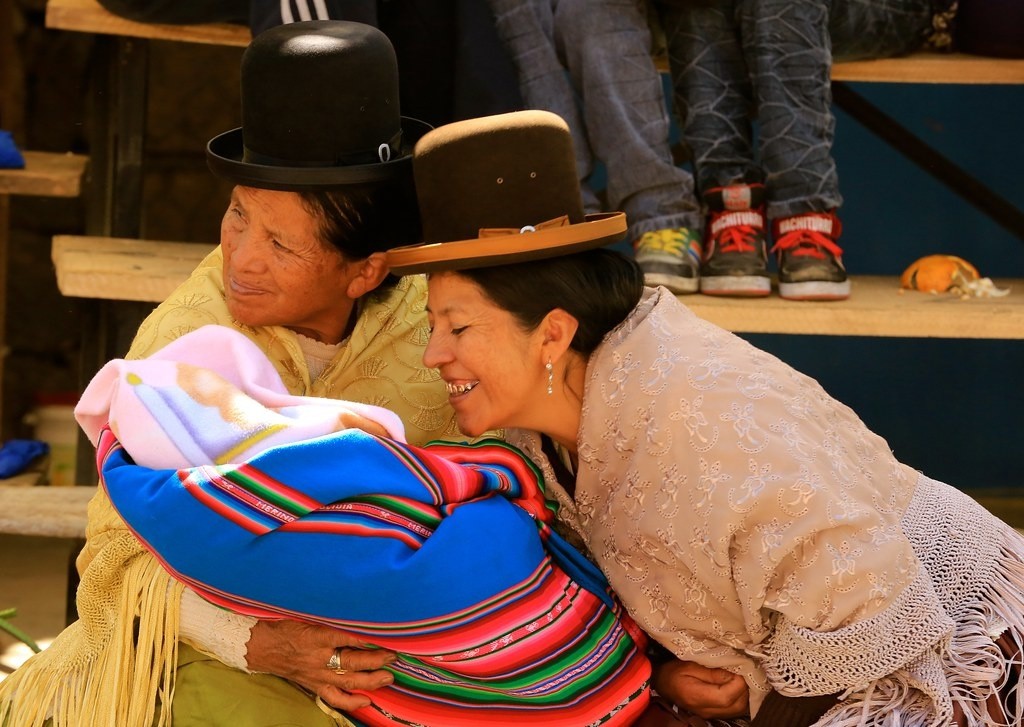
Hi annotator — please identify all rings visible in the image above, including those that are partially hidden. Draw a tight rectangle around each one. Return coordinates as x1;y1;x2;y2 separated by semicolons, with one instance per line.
327;649;347;675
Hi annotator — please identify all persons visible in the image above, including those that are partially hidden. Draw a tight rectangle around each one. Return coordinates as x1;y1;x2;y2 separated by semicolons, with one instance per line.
385;109;1024;727
252;0;523;128
491;0;704;293
663;0;930;299
0;19;504;727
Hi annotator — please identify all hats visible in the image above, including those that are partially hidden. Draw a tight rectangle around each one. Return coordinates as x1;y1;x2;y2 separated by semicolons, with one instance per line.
386;109;627;277
205;18;433;191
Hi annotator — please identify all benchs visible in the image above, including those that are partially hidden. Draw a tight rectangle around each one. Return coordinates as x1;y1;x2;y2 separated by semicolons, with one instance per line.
0;0;1024;635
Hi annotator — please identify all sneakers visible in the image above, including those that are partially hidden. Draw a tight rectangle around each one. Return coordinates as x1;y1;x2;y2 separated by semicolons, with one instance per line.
631;227;701;294
770;206;851;301
700;182;771;298
